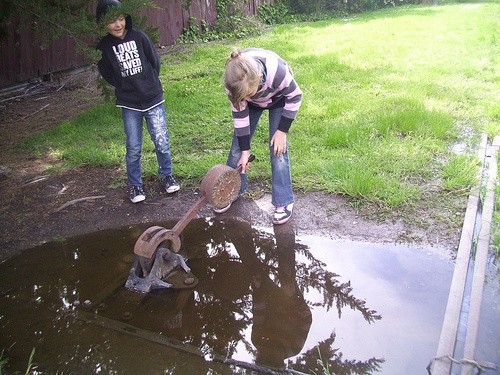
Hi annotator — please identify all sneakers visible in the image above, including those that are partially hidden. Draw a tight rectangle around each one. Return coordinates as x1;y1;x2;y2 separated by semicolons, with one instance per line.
163;176;180;193
129;186;145;202
213;192;244;212
273;202;294;224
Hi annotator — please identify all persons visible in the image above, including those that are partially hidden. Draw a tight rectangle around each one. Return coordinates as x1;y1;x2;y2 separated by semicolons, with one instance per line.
226;225;313;370
95;0;180;203
213;47;303;225
